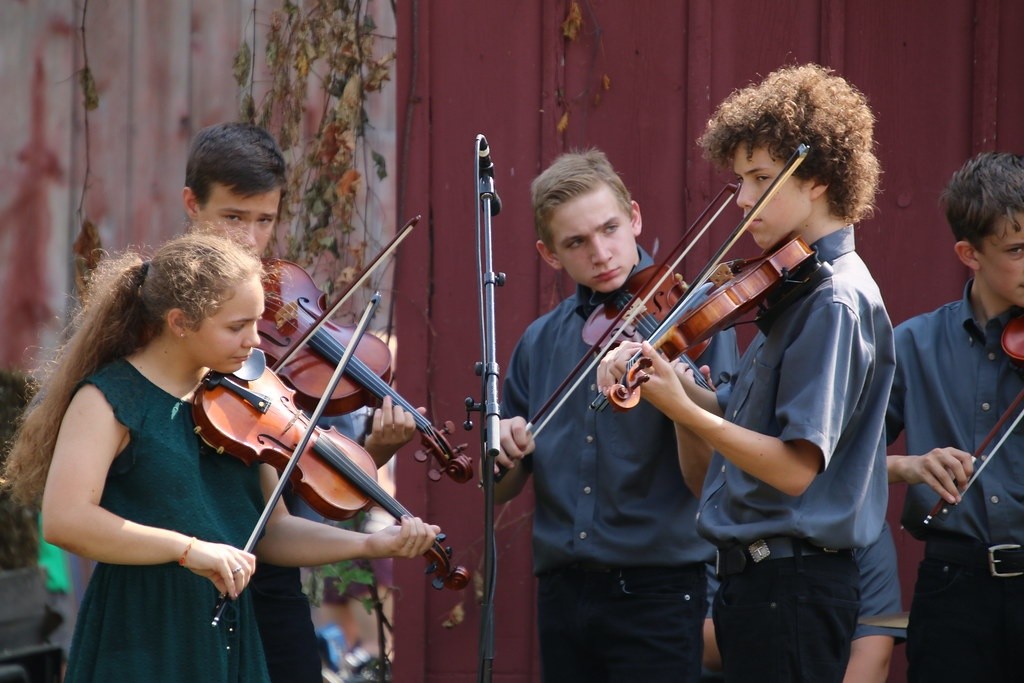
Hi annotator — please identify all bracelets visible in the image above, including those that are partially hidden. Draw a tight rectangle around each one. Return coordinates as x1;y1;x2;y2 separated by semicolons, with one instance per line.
179;536;197;567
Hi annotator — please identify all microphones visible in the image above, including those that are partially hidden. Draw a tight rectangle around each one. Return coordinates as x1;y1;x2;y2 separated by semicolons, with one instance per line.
477;136;502;216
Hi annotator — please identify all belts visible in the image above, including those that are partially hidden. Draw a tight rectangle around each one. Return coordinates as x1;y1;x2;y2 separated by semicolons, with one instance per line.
925;537;1024;577
716;535;826;579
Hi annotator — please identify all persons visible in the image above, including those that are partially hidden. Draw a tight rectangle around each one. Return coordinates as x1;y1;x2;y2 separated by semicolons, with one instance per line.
887;151;1018;682
597;61;907;683
4;121;443;682
481;142;741;682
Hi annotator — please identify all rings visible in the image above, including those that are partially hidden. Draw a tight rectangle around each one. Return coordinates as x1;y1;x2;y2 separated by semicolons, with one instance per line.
231;566;242;573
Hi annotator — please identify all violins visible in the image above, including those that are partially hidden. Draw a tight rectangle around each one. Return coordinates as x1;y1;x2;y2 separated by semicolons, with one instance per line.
593;236;821;414
192;353;473;592
583;258;719;391
254;261;476;483
997;312;1024;360
